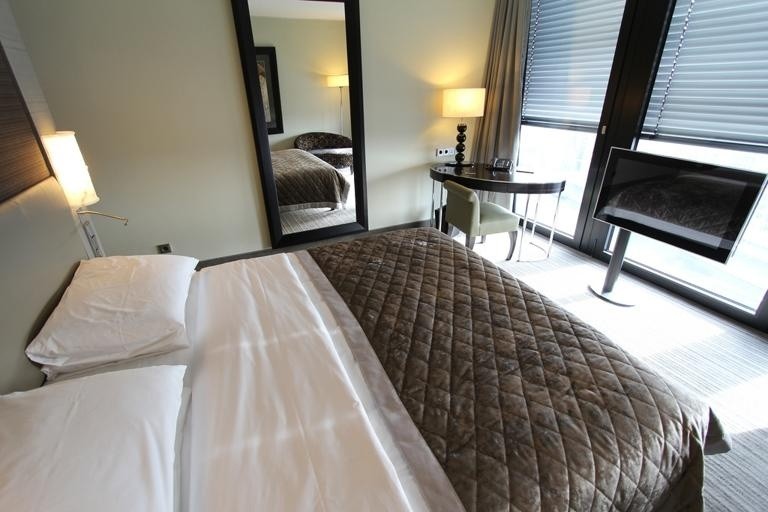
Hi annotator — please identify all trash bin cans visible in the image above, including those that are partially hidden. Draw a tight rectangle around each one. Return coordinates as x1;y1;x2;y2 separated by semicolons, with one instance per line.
435;205;459;237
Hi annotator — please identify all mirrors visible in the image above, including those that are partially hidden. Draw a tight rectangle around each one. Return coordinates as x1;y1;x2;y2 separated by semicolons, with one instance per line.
229;2;369;253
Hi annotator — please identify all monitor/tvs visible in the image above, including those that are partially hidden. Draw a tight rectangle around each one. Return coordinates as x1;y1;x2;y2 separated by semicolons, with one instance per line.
593;146;768;266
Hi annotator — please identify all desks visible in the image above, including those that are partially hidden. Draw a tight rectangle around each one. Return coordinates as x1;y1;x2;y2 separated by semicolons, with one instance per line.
430;161;566;263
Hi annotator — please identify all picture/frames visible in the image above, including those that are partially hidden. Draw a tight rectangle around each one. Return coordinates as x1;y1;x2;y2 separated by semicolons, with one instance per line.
254;46;284;135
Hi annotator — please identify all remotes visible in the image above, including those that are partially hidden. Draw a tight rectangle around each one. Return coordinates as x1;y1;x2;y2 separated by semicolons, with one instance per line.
445;162;474;166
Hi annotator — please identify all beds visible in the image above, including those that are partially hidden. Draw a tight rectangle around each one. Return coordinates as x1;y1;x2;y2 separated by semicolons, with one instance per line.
271;147;344;214
2;177;714;512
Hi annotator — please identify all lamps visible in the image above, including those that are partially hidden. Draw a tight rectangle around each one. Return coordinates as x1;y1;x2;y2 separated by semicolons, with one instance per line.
38;128;99;216
326;73;349;136
442;88;486;168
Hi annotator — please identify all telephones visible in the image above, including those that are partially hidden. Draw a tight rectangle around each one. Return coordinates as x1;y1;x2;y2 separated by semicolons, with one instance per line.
485;158;512;172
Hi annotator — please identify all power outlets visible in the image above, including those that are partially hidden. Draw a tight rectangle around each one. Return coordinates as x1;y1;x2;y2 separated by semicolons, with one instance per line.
158;243;171;254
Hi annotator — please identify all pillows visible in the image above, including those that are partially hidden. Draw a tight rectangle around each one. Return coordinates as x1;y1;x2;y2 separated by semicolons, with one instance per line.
5;364;191;512
26;256;199;370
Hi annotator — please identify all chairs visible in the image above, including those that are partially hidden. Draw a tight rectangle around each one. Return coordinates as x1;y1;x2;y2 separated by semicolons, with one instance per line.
442;178;521;261
294;132;352;175
